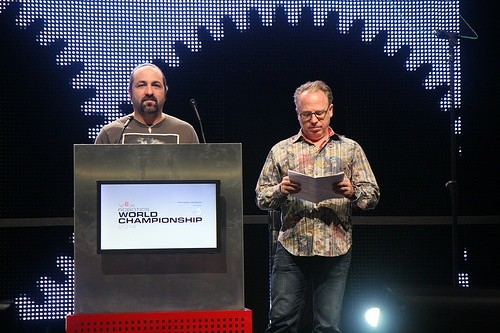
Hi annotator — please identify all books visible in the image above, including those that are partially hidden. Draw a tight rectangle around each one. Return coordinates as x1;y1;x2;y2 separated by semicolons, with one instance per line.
287;167;345;205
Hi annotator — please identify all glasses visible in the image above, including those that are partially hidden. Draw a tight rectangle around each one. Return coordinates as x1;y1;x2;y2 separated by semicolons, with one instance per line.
297;106;329;117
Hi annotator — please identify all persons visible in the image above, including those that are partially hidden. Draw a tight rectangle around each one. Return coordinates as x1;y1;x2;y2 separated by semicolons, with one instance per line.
93;63;199;144
255;80;380;333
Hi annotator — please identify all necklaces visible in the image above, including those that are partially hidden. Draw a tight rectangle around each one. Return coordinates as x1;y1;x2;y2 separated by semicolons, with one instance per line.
134;117;162;134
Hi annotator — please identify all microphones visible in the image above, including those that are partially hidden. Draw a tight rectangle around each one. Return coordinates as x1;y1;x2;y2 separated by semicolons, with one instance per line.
436;30;475;40
117;115;135;144
190;99;206;143
